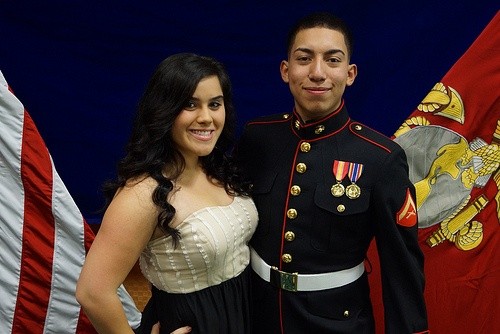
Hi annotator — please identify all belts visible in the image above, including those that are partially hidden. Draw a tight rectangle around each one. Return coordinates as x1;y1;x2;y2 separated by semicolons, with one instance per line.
249;248;367;293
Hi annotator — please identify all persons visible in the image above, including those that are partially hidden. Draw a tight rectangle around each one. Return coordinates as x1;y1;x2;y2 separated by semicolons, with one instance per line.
234;15;430;334
75;52;259;334
